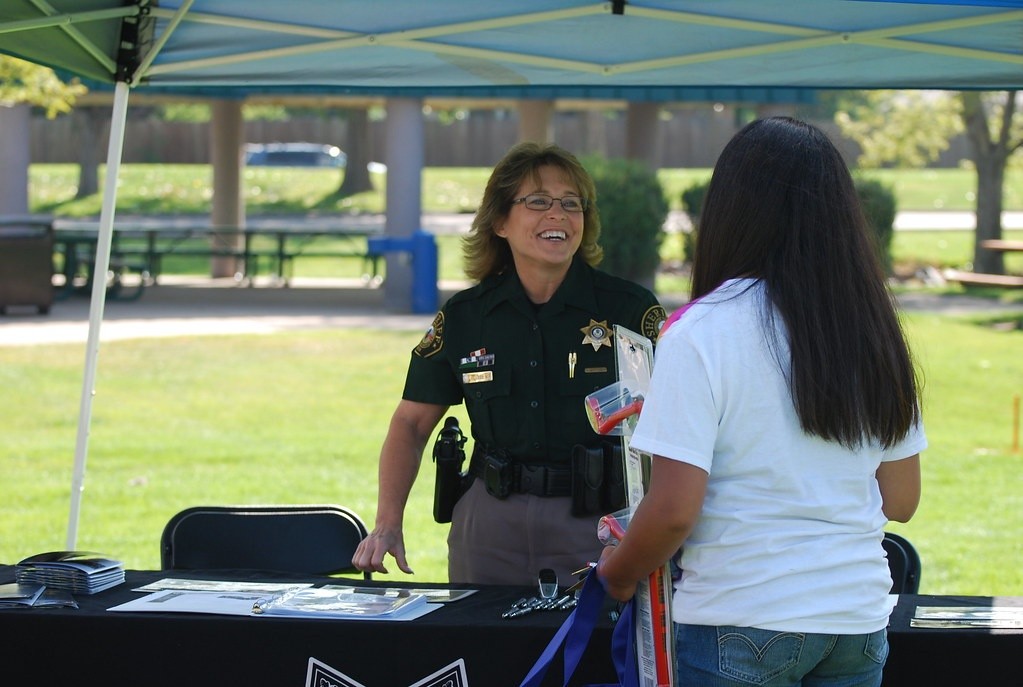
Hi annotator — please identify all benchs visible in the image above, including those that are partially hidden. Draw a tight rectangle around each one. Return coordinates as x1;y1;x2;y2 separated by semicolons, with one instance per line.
945;272;1023;289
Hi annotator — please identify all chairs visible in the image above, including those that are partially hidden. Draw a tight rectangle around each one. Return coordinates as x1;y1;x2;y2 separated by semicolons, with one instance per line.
160;506;372;580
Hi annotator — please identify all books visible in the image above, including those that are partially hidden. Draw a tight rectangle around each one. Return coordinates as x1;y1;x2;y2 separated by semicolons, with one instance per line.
0;551;126;611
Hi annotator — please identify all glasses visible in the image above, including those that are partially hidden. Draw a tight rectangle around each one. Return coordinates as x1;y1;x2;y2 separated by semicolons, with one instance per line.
511;193;587;212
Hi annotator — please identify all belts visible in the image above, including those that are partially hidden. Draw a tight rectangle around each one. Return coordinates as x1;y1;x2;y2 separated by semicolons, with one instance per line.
468;444;570;498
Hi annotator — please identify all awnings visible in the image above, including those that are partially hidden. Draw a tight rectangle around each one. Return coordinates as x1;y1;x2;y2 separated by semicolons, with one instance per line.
0;0;1023;551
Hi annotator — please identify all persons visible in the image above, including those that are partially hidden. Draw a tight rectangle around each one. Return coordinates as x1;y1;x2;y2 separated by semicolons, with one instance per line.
595;116;928;687
352;146;668;584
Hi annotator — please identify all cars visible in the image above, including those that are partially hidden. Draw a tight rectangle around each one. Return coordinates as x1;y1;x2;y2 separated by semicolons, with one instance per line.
246;140;387;176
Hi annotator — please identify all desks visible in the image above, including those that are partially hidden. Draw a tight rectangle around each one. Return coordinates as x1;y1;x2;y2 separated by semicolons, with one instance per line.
0;562;1023;686
980;239;1023;329
